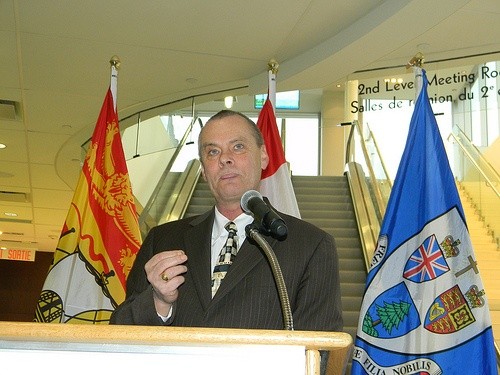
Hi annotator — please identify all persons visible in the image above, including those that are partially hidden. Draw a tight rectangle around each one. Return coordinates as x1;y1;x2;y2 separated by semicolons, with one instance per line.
107;110;344;375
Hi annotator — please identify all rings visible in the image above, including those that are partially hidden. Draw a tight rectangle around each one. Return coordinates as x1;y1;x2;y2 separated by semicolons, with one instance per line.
161;273;169;282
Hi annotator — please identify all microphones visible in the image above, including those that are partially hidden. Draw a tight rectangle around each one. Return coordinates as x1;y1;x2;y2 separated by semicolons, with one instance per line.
240;190;288;236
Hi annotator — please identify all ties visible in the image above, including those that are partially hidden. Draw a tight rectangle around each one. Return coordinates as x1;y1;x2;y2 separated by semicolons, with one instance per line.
210;222;239;298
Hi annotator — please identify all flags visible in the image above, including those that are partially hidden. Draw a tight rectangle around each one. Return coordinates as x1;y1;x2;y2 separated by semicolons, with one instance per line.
33;88;143;325
348;71;499;375
254;100;302;220
